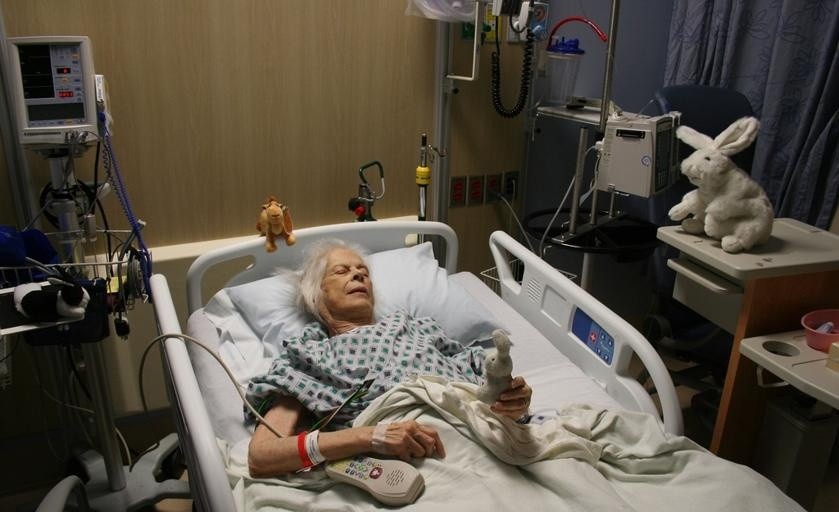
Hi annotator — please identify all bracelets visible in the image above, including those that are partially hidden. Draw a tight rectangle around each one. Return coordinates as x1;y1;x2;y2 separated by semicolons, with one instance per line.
298;429;326;468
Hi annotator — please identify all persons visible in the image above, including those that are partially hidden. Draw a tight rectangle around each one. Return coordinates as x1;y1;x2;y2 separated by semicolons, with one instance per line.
241;238;532;480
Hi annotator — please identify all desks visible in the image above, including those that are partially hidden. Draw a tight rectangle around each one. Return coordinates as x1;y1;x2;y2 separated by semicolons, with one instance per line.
739;329;839;410
657;218;839;464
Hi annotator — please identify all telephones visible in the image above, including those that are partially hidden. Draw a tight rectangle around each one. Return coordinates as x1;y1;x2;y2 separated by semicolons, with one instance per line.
492;0;521;16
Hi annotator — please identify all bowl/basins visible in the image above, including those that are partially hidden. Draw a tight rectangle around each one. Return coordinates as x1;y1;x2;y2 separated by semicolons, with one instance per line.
799;307;839;349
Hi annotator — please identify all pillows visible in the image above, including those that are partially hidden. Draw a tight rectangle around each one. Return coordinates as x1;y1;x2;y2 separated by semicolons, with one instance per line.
203;240;513;389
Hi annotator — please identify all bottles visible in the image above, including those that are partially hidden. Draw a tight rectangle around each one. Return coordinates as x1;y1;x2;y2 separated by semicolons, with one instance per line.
816;322;834;333
545;39;585;107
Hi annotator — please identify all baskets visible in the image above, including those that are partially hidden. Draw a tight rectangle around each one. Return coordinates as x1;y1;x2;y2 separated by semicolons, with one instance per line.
480;258;577;298
0;229;152;315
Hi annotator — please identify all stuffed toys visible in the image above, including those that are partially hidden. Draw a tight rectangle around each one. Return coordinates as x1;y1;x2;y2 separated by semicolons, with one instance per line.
666;114;774;253
12;276;91;323
255;196;296;253
476;330;513;405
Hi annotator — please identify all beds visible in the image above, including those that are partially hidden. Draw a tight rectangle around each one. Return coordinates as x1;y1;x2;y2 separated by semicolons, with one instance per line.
149;222;805;512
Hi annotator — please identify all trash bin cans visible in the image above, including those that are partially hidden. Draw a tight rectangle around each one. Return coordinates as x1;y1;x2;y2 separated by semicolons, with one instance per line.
691;390;719;450
